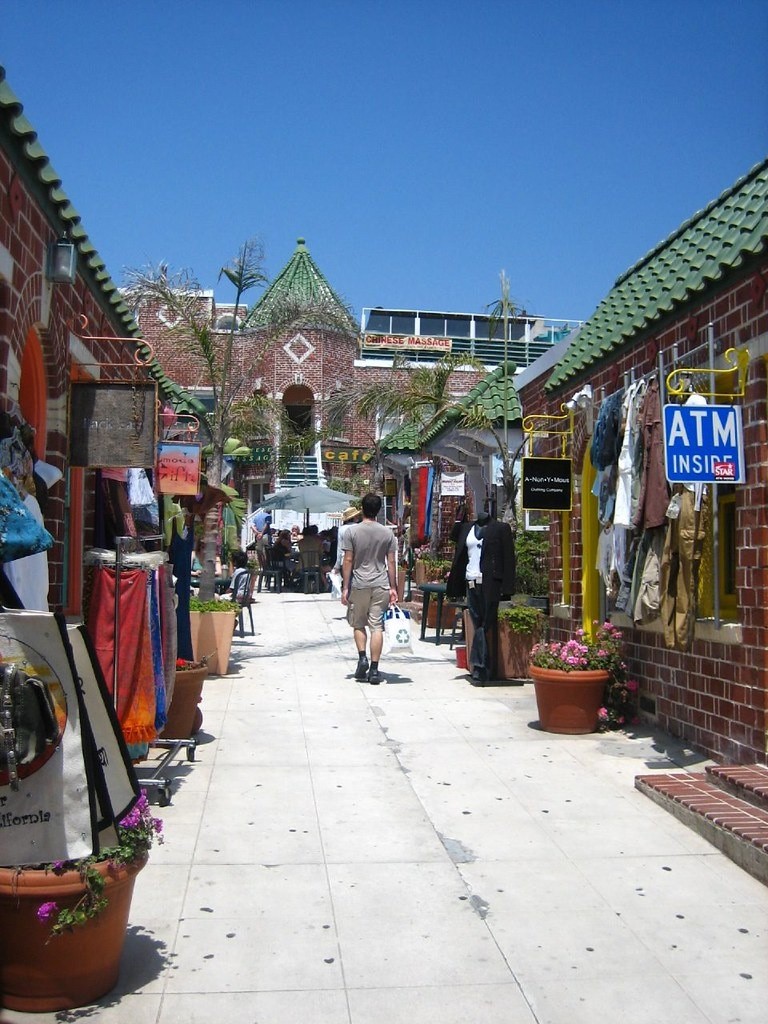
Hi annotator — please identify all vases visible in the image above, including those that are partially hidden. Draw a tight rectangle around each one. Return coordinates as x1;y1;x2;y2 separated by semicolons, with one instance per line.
0;848;149;1013
151;661;209;748
530;663;610;736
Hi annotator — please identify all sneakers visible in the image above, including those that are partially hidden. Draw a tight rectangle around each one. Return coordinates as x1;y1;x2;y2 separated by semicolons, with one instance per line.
356;656;368;678
368;670;384;683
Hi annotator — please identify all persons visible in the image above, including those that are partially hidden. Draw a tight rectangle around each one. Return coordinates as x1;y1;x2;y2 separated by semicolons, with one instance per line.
271;525;338;591
333;504;362;589
250;508;272;589
341;492;399;686
188;536;222;577
445;511;515;680
215;551;251;631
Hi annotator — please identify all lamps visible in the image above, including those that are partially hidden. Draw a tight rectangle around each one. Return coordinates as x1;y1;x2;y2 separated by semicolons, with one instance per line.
563;384;594;412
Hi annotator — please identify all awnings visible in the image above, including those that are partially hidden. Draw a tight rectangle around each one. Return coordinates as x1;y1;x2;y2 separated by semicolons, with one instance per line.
255;480;358;524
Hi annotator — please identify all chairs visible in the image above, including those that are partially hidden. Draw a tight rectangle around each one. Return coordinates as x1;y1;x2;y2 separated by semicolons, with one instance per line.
299;550;322;594
256;544;286;594
231;571;255;638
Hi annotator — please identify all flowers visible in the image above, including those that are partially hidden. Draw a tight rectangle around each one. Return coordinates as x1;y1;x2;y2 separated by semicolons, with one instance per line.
528;621;639;728
176;657;196;672
38;787;165;946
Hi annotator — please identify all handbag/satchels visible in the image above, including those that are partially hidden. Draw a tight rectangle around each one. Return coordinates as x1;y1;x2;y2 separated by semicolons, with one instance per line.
380;601;415;650
